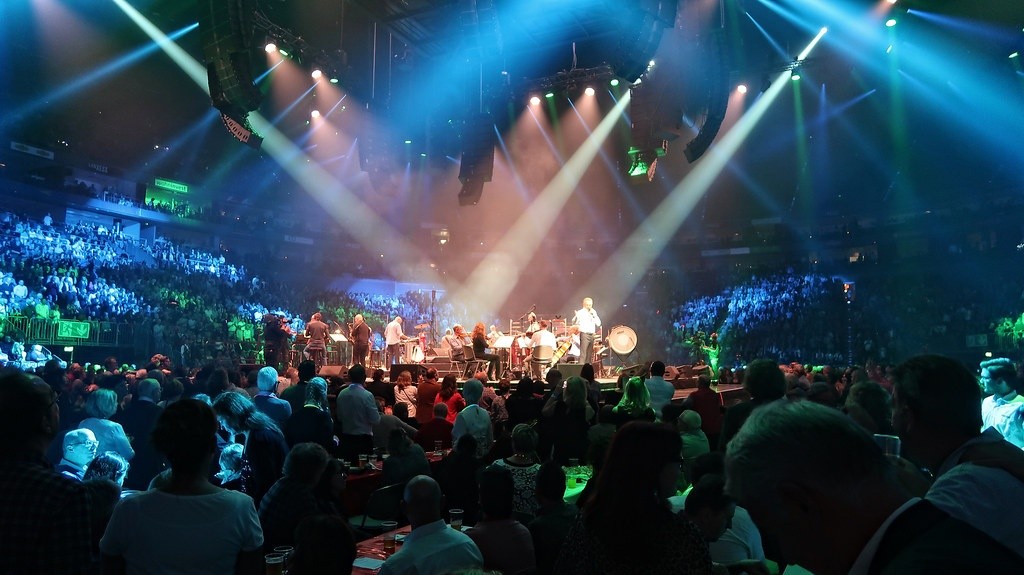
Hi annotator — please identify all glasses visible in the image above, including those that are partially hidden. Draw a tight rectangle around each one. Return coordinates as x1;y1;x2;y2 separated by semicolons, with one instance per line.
665;457;689;473
49;391;63;409
70;441;99;448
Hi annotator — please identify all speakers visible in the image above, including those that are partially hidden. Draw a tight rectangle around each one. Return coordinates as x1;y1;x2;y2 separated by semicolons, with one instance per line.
622;365;644;378
390;364;428;383
552;363;583;381
319;366;348;379
239;363;266;374
663;365;711;380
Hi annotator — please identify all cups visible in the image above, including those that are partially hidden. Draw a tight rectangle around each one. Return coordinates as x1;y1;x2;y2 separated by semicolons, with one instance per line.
265;553;284;575
449;508;464;532
382;454;390;469
358;454;367;468
368;455;378;468
273;545;294;575
568;474;576;488
380;521;397;549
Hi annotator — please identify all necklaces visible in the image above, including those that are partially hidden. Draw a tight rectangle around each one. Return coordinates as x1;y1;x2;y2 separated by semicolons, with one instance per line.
515;451;534;462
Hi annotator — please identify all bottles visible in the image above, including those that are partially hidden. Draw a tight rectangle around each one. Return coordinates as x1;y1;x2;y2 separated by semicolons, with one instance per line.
338;458;351;471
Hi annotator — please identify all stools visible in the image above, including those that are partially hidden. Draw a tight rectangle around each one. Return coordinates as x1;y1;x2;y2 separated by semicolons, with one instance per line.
290;342;380;370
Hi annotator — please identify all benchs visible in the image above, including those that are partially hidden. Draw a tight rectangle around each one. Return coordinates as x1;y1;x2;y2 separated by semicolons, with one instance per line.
351;524;473;575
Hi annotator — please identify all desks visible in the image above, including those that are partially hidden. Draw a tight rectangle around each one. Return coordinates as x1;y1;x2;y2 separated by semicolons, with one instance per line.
562;466;592;504
342;447;454;518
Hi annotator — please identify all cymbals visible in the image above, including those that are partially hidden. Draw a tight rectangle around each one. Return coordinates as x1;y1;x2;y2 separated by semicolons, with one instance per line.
414;324;429;328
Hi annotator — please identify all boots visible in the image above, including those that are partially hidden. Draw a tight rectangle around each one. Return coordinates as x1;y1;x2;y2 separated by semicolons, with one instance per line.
488;371;495;381
496;372;503;381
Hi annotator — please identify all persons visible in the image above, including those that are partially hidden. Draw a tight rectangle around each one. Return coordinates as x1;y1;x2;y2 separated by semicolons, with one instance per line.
0;179;1024;575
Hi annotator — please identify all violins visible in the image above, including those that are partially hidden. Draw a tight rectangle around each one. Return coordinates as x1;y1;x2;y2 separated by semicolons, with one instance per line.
460;332;472;338
485;334;495;341
513;331;535;340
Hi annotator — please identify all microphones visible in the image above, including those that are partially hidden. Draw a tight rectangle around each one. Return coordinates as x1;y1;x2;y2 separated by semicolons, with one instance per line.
589;308;595;318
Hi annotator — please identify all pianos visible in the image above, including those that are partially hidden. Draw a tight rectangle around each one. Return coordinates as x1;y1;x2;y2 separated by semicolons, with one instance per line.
292;335;330;367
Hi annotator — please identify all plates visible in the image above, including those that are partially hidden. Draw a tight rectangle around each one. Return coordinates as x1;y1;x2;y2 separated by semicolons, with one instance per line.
395;533;408;542
352;556;386;570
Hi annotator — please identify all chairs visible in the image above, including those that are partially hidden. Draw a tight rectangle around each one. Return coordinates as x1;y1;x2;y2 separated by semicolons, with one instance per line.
462;345;490;381
448;350;464;378
525;345;553;381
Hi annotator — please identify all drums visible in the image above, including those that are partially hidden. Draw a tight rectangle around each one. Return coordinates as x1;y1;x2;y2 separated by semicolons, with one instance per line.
609;326;637;355
